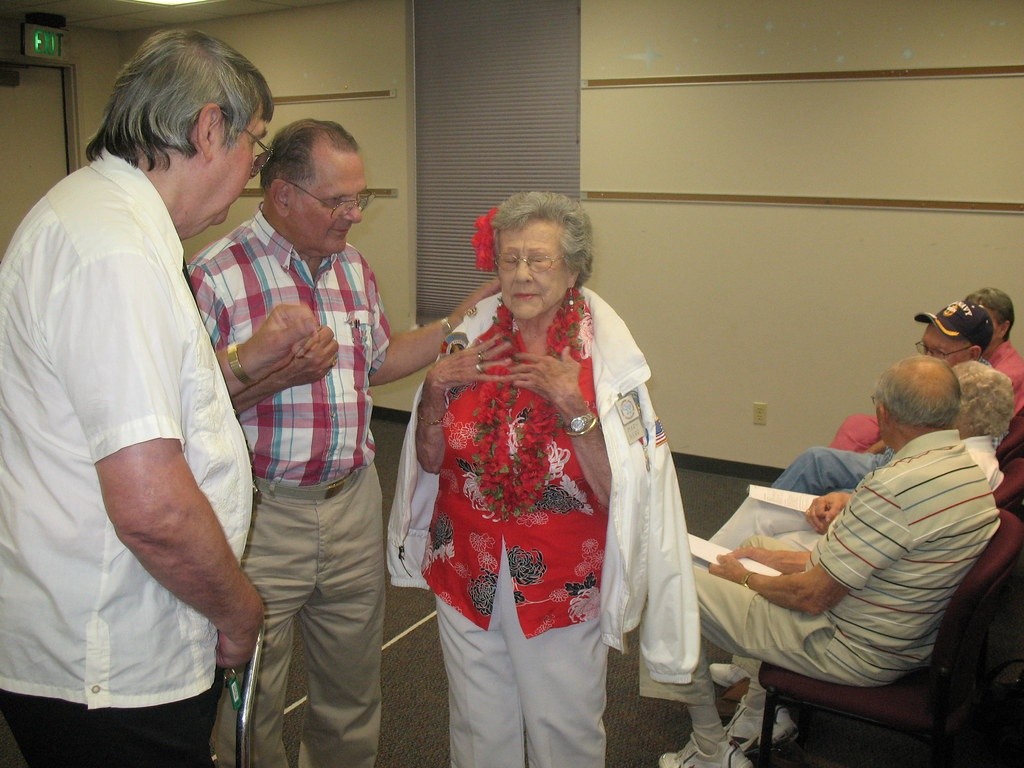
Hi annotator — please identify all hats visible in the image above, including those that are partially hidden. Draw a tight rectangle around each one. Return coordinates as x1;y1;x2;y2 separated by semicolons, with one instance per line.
914;301;993;353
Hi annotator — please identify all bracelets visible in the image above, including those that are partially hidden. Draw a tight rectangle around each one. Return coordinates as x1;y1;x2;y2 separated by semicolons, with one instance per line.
740;572;759;589
227;342;258;387
417;411;444;425
566;415;598;437
441;316;453;336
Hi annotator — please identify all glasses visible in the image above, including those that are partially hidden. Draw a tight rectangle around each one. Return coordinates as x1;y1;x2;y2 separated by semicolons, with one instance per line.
915;341;976;360
494;251;567;273
220;110;273;176
870;393;890;416
279;178;374;221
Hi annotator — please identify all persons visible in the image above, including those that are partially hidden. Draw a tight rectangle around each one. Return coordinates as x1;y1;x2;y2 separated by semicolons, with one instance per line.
183;117;503;768
771;299;994;496
827;286;1024;455
709;359;1015;687
659;354;1000;768
386;188;700;768
1;25;321;768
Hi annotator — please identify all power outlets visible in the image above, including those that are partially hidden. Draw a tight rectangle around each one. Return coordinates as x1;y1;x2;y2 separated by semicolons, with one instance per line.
753;402;768;425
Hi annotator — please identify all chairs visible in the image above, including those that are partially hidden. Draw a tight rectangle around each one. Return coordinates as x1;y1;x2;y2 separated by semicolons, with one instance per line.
757;407;1024;768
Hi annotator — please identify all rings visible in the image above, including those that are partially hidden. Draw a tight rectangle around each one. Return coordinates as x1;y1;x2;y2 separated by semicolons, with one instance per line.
478;349;486;362
474;363;484;372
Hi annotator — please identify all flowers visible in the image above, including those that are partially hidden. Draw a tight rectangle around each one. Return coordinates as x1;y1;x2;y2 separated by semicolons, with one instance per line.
471;208;498;271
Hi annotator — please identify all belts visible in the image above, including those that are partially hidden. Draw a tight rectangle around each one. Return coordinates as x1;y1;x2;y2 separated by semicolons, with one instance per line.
251;470;356;500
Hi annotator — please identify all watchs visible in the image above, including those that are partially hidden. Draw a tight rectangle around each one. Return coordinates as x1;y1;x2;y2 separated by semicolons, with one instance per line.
564;411;595;432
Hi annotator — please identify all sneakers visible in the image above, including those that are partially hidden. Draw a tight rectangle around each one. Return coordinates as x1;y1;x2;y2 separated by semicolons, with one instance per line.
709;662;749;688
659;733;753;768
724;696;799;755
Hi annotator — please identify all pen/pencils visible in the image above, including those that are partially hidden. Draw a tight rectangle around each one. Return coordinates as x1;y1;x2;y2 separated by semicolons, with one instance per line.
354;319;360;328
